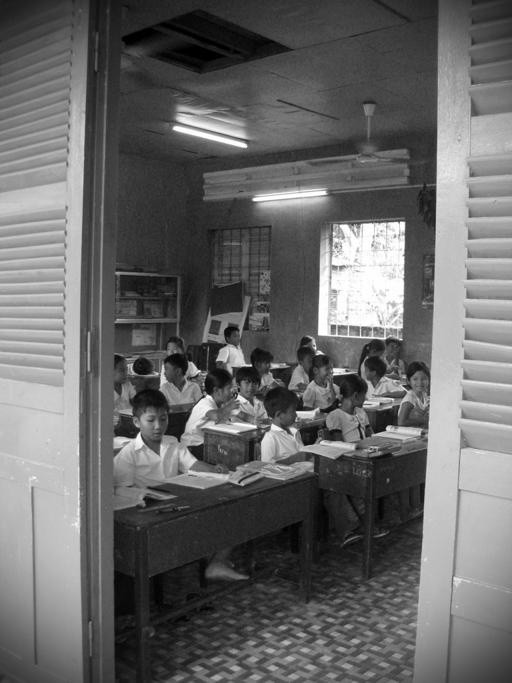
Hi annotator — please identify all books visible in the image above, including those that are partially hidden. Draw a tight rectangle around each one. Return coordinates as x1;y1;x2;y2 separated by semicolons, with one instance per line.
125;291;140;296
164;470;232;489
301;439;359;460
118;300;137;317
113;435;134;450
334;367;348;374
113;487;179;512
237;461;305;480
249;301;270;331
206;421;256;434
364;397;393;408
118;408;134;415
145;304;163;318
295;408;320;421
229;469;263;486
371;424;424;442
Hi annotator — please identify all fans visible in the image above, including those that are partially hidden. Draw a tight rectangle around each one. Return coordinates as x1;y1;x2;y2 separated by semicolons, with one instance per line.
306;102;433;172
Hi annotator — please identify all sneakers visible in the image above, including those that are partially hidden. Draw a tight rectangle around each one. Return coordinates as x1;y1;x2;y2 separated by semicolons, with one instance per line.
341;532;363;549
372;527;390;538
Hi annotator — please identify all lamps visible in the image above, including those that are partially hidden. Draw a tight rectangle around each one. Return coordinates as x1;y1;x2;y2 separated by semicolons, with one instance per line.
252;188;329;202
173;125;248;150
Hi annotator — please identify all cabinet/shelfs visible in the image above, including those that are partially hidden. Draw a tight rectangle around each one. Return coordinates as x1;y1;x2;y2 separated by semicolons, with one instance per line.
116;271;182;354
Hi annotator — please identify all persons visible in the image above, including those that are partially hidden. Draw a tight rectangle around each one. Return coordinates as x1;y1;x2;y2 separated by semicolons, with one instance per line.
112;390;250;582
180;369;240;460
261;388;390;548
363;356;409;397
215;327;246;377
162;337;202;379
232;348;286;400
326;375;376;444
299;334;324;357
160;354;203;405
301;354;341;412
397;360;431;515
222;369;273;425
112;355;137;410
385;336;407;374
127;356;160;378
357;339;389;388
288;346;316;390
